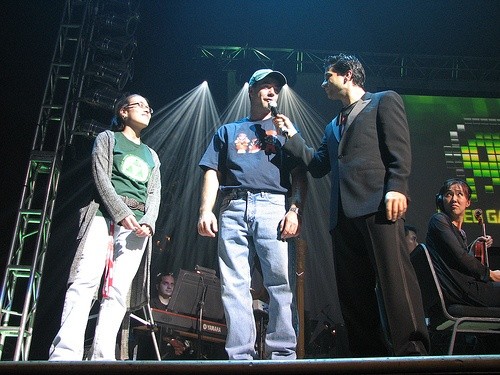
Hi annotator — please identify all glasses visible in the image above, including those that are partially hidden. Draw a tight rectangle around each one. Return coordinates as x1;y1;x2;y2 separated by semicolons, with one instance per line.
122;102;153;113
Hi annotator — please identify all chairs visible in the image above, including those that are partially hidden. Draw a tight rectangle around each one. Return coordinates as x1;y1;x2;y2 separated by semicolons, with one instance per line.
409;243;500;356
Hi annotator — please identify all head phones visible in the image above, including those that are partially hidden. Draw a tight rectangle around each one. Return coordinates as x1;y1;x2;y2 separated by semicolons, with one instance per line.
435;179;473;208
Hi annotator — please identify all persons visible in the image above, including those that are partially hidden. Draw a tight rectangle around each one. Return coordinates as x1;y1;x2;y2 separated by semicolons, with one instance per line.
427;178;500;307
50;95;161;361
273;52;431;355
137;273;207;359
197;69;304;360
405;225;419;254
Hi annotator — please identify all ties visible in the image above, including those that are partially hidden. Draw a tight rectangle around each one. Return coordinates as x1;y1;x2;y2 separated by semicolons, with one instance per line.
339;105;353;136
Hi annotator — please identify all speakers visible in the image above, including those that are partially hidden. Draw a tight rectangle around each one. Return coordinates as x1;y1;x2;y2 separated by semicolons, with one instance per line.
202;277;226;321
167;269;204;316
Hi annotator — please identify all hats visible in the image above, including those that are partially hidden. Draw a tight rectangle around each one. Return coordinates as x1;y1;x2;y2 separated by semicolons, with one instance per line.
249;69;287;87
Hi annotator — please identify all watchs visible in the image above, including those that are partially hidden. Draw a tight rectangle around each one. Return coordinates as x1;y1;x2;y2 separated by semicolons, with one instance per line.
290;207;303;217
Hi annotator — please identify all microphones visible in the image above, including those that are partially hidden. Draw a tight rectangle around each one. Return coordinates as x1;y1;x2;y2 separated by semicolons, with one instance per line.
268;101;288;135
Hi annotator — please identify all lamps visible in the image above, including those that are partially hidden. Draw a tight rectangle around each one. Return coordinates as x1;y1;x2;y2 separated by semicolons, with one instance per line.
101;0;141;14
89;59;130;92
88;35;135;62
74;117;115;139
94;11;140;39
78;88;128;115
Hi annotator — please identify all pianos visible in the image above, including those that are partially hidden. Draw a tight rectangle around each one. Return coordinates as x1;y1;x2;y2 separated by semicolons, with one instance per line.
130;304;228;359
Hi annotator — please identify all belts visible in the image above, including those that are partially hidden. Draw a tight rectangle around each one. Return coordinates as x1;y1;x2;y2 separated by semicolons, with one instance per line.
118;195;144;212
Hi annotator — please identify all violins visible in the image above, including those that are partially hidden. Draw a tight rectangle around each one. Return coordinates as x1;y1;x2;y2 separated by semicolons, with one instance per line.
474;208;489;268
